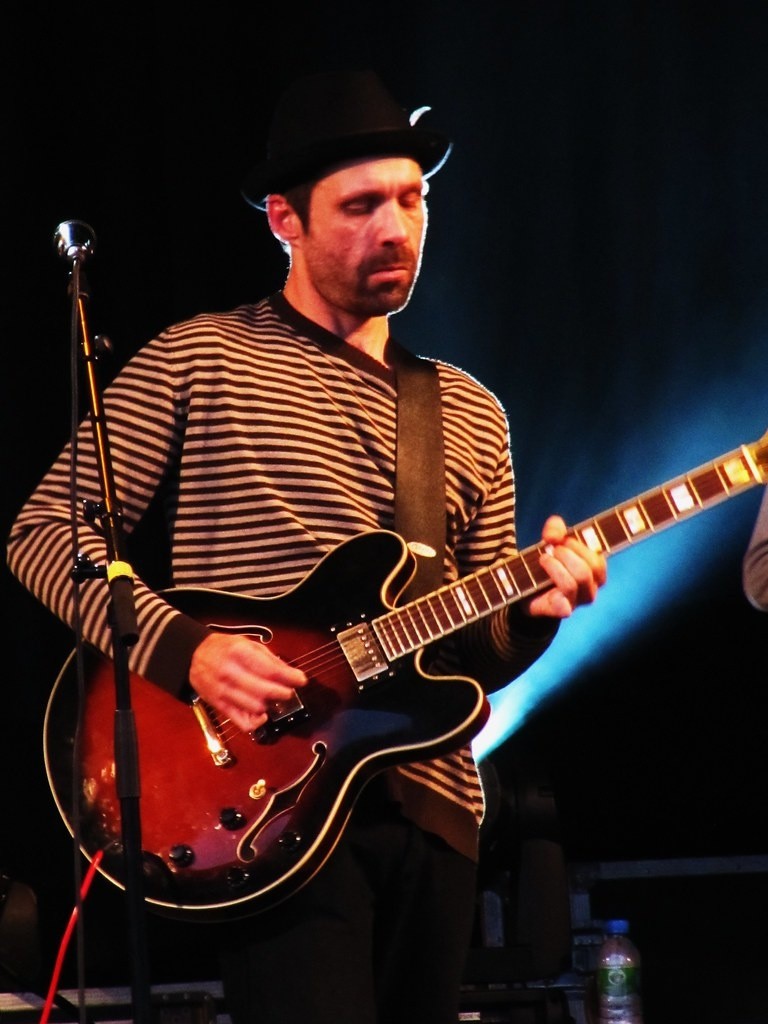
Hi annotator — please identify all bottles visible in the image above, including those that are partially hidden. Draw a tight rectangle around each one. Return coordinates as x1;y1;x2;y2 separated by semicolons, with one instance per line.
591;920;643;1024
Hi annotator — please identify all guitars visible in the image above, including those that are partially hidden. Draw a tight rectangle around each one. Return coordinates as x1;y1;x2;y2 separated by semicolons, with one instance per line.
44;433;768;910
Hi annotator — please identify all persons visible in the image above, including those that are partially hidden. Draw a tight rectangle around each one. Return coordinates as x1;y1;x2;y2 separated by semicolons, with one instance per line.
747;477;766;612
3;73;609;1023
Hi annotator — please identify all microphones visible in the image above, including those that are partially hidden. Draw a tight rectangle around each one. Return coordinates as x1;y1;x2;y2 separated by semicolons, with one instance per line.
54;220;97;262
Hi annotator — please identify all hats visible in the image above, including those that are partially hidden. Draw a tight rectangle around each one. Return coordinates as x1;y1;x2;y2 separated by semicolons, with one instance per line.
239;70;454;212
